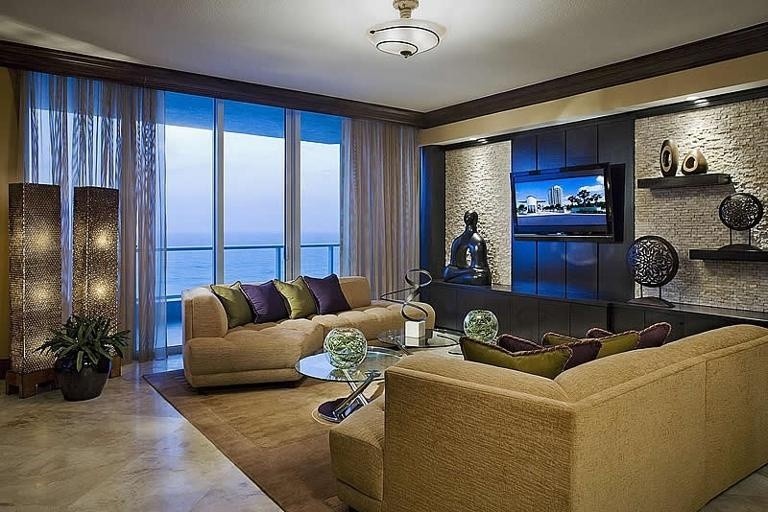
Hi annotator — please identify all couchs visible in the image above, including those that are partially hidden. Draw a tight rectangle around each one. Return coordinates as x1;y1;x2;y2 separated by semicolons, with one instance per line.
180;274;435;393
328;321;768;512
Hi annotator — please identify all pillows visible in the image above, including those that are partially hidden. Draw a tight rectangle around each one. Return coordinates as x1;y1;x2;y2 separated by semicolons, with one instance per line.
239;279;291;323
585;321;671;349
457;335;579;382
272;275;319;319
497;333;602;371
210;281;256;329
540;330;640;357
304;274;352;314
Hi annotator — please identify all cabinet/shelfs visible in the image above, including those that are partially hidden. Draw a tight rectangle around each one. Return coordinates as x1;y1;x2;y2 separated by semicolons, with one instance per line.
637;173;768;262
420;277;608;345
609;299;768;345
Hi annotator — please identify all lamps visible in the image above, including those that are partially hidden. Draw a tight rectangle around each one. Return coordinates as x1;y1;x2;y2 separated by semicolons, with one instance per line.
6;182;64;398
72;187;122;379
366;0;446;59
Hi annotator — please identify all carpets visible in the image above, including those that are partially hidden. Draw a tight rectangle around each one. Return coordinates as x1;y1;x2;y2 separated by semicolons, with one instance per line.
142;350;768;512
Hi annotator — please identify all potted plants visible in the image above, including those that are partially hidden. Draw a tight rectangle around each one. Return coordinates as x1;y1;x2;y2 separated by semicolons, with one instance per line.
31;314;131;401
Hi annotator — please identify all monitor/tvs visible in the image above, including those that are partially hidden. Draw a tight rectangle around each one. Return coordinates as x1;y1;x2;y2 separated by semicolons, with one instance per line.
510;162;615;243
